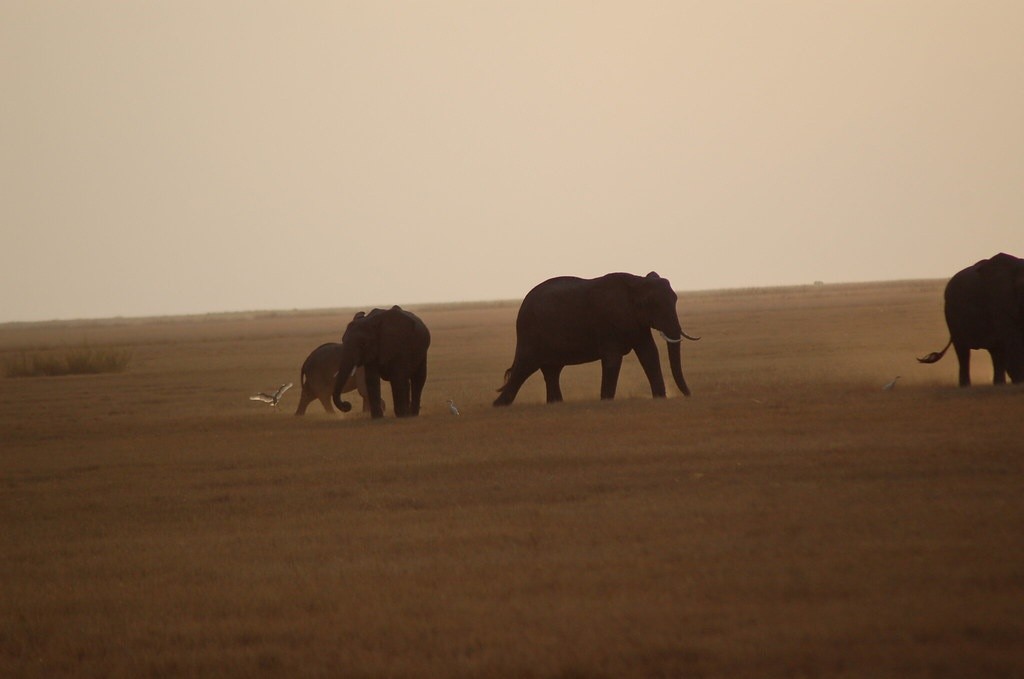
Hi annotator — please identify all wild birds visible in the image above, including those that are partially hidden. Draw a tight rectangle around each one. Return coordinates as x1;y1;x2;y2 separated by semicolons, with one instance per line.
248;383;294;408
447;399;460;416
882;376;899;391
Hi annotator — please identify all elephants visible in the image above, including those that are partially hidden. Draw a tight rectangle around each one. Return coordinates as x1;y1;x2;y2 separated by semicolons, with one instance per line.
295;305;431;418
917;252;1024;388
493;271;702;407
